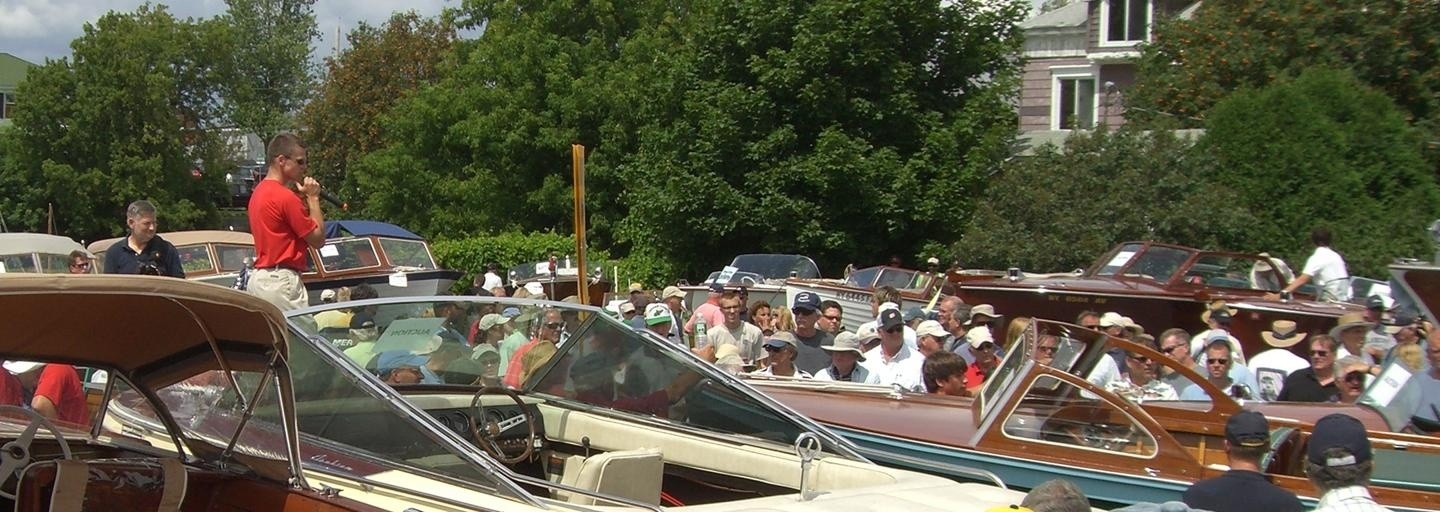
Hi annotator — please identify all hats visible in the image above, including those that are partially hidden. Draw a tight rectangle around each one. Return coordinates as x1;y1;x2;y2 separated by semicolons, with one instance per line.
1366;295;1385;309
1226;410;1270;449
347;312;375;333
1262;320;1307;348
1329;313;1377;339
1254;252;1273;272
1100;312;1144;335
1201;300;1238;324
320;289;336;303
762;292;866;362
1206;329;1227;346
620;282;688;326
523;282;543;295
376;349;432;377
708;283;748;295
856;301;1003;350
471;307;562;385
1307;413;1371;467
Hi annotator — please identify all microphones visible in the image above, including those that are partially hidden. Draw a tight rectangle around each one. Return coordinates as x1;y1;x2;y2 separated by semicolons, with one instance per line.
300;175;348;211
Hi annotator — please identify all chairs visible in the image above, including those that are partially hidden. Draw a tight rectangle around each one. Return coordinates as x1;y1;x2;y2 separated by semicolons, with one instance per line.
563;447;666;509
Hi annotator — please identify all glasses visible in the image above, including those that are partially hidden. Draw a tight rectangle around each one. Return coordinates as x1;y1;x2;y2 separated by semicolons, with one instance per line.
1345;374;1366;382
1308;350;1326;356
274;154;305;165
1127;343;1227;364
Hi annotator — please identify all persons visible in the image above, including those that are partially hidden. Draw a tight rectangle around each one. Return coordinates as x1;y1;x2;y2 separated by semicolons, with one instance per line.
1181;409;1304;512
66;250;91;274
1300;413;1393;512
312;226;1439;438
30;363;92;435
1;355;25;410
104;200;185;278
182;251;192;263
990;479;1093;511
245;134;325;314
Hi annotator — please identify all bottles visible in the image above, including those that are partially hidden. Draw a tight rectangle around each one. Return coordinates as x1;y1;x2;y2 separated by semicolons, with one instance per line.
693;311;708;350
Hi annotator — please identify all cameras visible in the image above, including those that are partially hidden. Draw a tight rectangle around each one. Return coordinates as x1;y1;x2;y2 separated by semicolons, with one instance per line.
1416;313;1432;340
